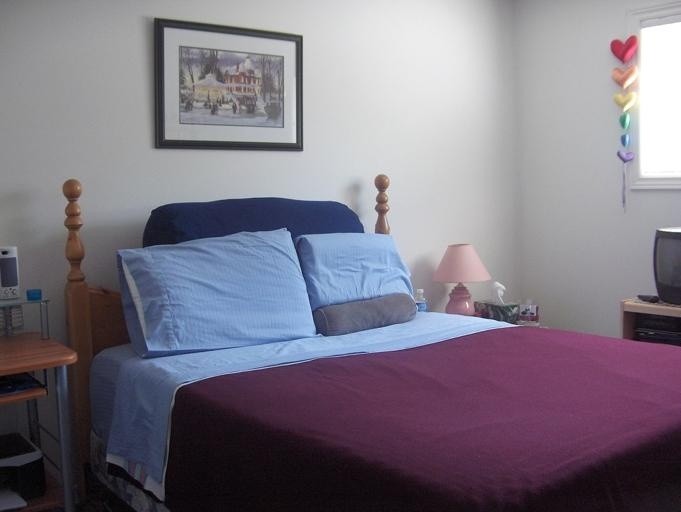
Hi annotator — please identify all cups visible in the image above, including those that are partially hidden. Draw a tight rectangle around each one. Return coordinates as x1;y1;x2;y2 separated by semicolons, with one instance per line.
26;288;42;301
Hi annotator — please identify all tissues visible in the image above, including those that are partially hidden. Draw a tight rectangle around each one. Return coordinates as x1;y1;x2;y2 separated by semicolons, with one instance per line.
473;282;521;323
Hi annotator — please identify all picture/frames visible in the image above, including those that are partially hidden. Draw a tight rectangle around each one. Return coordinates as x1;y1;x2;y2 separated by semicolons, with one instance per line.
149;15;306;154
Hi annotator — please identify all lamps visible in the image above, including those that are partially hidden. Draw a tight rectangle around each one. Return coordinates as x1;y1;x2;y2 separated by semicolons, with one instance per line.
433;240;494;316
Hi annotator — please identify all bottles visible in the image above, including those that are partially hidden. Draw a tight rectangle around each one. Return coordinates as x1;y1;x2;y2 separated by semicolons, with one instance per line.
415;288;427;312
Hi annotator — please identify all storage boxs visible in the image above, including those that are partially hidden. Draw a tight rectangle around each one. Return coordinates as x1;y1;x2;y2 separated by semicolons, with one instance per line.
0;430;50;504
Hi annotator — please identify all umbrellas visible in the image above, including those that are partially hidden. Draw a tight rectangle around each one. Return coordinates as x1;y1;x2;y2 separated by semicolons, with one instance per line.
193;74;225;100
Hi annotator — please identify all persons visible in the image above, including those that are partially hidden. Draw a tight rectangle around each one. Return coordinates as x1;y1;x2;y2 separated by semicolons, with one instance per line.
180;94;238;116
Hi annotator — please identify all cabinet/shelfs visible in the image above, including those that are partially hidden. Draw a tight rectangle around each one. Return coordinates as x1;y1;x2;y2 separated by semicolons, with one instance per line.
618;294;681;352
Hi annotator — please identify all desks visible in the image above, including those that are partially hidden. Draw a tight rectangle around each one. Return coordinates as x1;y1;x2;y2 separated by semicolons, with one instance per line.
0;330;75;512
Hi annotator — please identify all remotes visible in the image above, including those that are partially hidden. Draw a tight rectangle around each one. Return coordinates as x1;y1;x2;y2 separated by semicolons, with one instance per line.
637;294;658;303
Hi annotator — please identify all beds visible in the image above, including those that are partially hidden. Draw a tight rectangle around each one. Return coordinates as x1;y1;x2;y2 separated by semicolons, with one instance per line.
56;162;680;512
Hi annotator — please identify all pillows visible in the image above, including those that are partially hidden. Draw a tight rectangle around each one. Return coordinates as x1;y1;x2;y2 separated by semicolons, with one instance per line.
117;223;320;360
292;231;415;309
313;289;421;337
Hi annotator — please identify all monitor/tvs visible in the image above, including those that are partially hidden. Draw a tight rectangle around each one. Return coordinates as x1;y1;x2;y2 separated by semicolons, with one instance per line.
653;228;681;304
